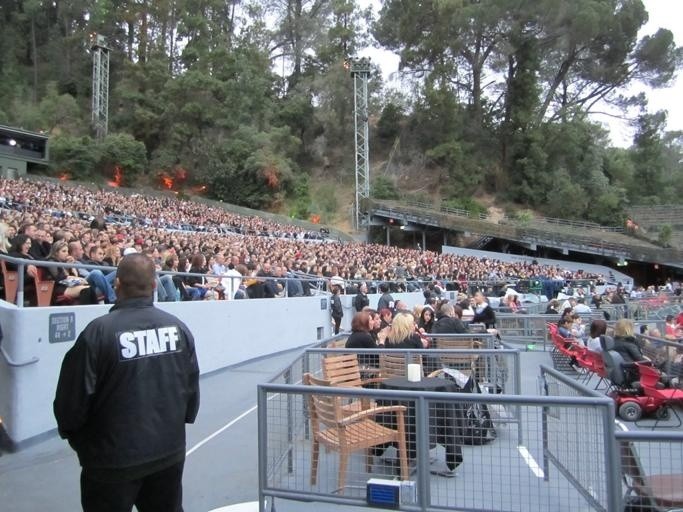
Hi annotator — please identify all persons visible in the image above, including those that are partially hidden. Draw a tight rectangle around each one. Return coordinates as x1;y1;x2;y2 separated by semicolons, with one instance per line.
329;235;682;314
51;253;200;511
331;285;501;377
557;308;683;388
0;169;328;308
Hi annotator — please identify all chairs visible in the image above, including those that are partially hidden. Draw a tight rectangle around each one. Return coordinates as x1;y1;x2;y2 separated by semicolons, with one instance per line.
545;322;683;432
304;316;482;495
614;418;683;512
